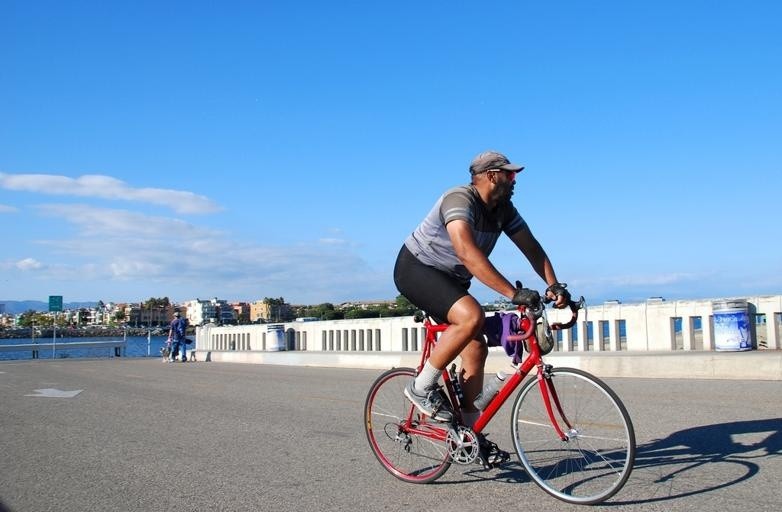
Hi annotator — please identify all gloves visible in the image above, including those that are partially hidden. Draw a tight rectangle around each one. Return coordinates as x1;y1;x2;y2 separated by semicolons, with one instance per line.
511;280;540;309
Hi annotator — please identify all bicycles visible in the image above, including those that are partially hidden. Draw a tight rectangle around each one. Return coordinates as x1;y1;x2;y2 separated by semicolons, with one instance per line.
362;282;637;506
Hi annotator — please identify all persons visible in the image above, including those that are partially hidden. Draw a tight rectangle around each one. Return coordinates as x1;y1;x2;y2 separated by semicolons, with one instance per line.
166;311;187;363
394;150;571;453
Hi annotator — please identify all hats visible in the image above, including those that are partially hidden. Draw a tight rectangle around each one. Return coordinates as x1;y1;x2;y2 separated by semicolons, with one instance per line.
172;312;179;317
469;151;525;175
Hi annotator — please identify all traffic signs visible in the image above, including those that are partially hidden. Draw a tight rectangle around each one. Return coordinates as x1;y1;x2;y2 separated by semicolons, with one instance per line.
48;295;63;312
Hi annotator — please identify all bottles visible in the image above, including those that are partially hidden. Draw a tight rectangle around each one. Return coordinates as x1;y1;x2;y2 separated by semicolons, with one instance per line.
473;370;507;410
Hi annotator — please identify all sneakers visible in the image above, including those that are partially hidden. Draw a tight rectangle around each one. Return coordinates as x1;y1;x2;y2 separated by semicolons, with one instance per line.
475;439;510;464
404;381;454;422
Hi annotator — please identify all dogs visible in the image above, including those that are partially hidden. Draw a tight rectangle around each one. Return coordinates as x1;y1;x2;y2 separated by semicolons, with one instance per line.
159;346;171;363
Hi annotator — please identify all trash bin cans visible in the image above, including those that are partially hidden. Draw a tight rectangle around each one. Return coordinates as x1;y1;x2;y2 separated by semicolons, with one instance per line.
713;298;753;352
266;325;286;351
